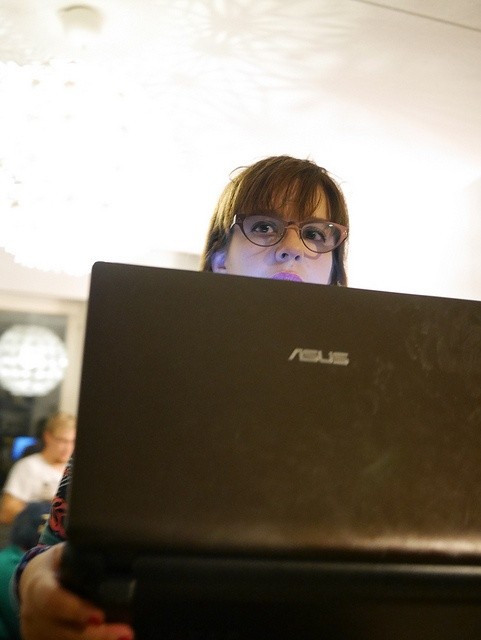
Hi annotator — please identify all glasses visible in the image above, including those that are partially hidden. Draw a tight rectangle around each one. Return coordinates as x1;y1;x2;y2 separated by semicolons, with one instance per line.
230;213;349;253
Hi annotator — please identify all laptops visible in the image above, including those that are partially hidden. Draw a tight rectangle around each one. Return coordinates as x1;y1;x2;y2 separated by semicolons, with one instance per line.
66;262;481;583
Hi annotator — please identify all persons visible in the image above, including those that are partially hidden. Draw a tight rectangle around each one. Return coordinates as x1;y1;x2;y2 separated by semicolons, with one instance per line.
0;413;77;550
1;156;353;634
